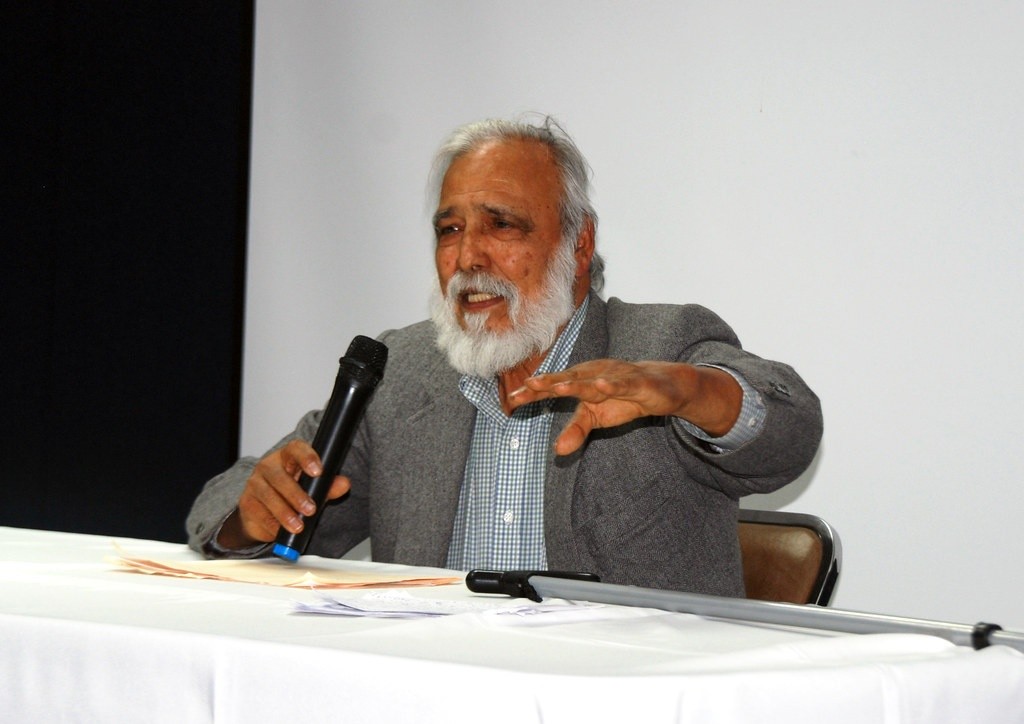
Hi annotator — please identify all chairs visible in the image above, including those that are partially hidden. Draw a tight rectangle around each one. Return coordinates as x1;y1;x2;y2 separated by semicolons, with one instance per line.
738;510;844;609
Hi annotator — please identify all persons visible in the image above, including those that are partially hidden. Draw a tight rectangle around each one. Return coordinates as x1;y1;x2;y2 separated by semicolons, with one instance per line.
185;118;825;601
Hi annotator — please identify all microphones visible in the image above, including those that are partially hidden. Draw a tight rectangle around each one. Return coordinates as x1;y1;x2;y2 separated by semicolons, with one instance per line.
272;335;389;562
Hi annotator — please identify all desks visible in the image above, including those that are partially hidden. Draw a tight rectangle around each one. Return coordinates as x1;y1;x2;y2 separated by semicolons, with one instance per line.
1;522;1024;724
